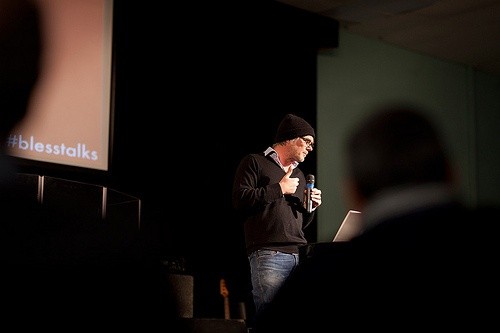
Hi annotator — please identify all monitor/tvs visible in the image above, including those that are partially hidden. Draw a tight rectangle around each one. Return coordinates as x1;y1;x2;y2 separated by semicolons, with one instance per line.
333;208;363;241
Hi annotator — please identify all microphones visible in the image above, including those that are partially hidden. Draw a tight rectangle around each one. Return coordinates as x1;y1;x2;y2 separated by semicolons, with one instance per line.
306;174;315;212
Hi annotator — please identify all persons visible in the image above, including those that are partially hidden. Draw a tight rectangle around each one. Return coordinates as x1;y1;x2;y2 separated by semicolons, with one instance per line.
258;105;500;333
231;114;322;310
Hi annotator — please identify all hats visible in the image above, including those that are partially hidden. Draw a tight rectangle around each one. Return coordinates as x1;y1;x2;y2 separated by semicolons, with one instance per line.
274;114;316;143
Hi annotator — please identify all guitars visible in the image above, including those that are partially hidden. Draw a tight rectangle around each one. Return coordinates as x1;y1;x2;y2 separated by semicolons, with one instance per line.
220;278;231;319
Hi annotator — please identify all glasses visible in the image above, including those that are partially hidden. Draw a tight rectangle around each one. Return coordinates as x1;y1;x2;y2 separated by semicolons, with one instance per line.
302;137;315;148
